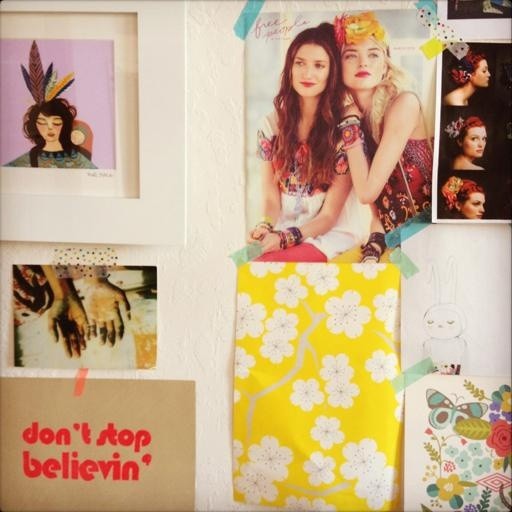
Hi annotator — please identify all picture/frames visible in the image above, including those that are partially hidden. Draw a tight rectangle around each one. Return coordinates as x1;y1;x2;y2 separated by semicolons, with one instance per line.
1;0;188;249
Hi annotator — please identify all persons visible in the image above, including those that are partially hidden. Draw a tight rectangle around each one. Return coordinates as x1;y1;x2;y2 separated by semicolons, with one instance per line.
39;265;132;361
